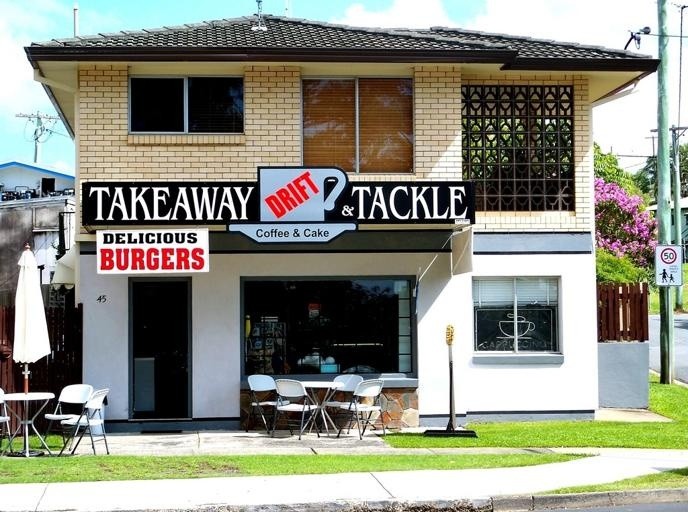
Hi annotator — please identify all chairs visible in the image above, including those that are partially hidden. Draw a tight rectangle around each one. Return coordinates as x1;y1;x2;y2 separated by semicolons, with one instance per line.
0;384;112;456
243;369;391;440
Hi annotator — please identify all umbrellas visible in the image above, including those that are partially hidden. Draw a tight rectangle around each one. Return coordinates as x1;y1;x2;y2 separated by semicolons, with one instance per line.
12;242;52;456
